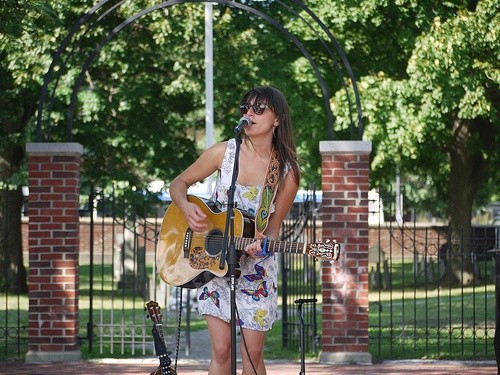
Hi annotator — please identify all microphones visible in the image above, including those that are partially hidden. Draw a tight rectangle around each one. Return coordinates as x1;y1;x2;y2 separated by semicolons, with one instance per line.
234;116;252;134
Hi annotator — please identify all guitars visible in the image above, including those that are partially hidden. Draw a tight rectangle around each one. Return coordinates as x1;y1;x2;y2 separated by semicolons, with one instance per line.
144;300;178;375
158;194;341;287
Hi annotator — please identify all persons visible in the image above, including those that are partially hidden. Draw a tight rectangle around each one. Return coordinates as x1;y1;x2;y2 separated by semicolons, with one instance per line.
169;86;301;375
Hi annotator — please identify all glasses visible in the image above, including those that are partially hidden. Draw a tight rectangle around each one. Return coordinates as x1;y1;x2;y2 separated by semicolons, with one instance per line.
238;102;267;115
